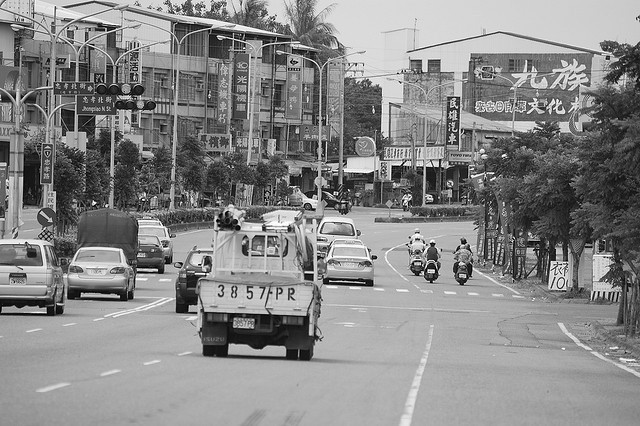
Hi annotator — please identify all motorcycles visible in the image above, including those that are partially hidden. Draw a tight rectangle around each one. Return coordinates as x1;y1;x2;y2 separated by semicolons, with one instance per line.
339;198;349;214
406;244;428;276
422;249;442;283
402;198;412;211
452;252;473;285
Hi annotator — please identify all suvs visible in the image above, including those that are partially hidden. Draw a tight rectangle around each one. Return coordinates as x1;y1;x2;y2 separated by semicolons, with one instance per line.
138;225;176;264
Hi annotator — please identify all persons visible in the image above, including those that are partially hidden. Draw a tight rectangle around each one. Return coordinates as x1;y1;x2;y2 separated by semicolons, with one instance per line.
456;237;473;255
407;237;427;269
422;239;442;276
402;191;412;207
453;243;472;278
339;191;352;211
409;228;425;245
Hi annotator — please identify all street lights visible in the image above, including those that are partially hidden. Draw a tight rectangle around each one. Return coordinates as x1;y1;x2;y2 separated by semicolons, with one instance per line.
10;23;142;154
479;149;489;265
386;78;468;206
62;37;171;208
216;35;300;167
0;3;129;143
26;102;76;230
0;86;53;239
124;18;236;209
276;50;366;210
477;69;556;138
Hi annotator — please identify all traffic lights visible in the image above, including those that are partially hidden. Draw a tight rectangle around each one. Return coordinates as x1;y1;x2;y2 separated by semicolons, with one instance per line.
96;83;144;95
115;99;156;111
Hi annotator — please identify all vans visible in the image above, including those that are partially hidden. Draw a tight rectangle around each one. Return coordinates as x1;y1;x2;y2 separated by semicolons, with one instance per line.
0;239;64;315
317;217;361;256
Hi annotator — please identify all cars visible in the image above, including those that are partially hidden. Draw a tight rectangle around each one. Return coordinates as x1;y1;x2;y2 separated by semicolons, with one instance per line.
136;234;166;273
277;192;318;210
304;191;348;210
174;245;213;313
67;247;135;301
323;239;371;252
320;244;377;286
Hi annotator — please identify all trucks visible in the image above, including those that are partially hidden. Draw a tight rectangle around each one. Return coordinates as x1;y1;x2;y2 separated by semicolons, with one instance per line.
78;208;140;276
195;222;324;360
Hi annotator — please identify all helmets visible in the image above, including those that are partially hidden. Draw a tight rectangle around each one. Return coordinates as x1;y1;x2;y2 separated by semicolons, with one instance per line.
429;240;436;244
415;235;421;240
460;237;467;244
465;244;470;249
415;228;420;234
